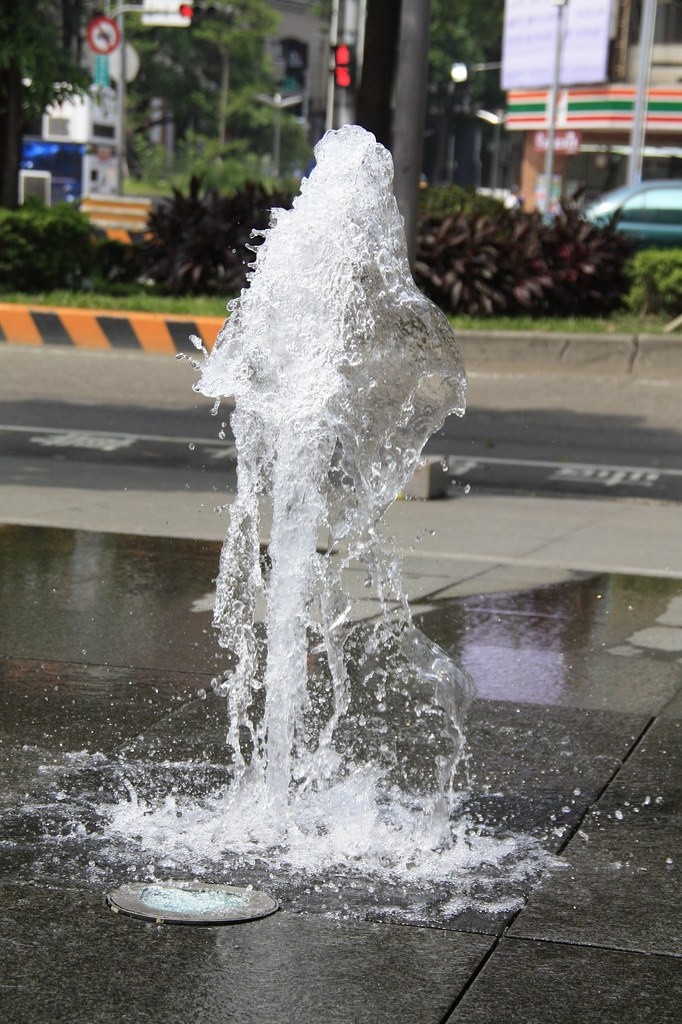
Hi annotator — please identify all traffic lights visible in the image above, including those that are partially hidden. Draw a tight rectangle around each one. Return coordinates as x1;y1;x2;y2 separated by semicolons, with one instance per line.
332;43;358;90
178;5;234;23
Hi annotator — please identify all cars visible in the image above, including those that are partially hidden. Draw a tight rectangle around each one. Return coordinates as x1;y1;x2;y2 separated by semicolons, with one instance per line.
579;176;682;252
